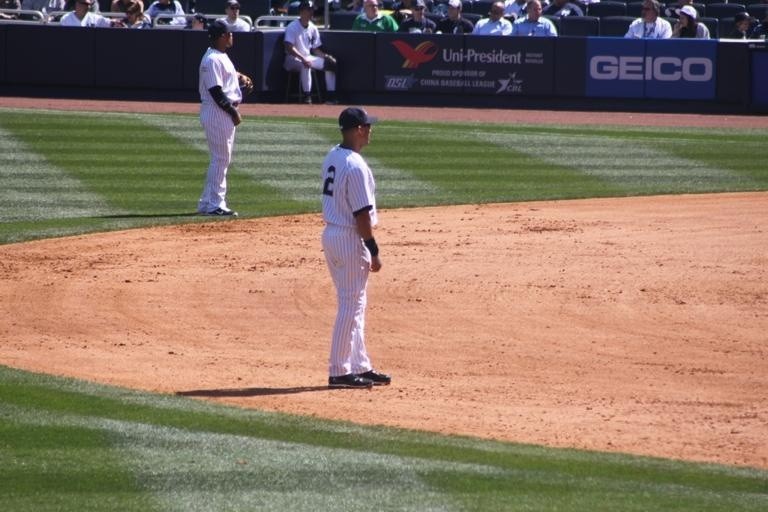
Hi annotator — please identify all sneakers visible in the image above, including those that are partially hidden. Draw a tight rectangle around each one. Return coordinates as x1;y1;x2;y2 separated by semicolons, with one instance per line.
327;375;375;390
356;372;391;386
211;207;240;218
304;94;312;104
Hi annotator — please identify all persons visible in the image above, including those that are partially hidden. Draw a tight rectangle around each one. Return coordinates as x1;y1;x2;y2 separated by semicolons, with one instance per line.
196;21;254;219
318;107;393;392
283;2;343;106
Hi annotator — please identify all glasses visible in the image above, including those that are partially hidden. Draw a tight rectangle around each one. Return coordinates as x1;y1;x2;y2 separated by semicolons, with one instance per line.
231;7;239;10
639;6;651;11
488;10;499;15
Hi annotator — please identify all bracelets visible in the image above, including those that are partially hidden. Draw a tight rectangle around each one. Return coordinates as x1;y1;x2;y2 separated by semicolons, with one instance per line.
364;238;379;257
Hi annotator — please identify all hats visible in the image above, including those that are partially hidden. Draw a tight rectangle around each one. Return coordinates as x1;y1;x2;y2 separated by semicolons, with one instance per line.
207;20;240;33
675;4;697;20
300;0;317;9
337;106;378;130
448;0;464;10
413;1;426;8
734;12;752;23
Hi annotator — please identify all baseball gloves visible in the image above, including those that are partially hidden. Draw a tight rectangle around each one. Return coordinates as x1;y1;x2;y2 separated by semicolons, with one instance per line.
239;74;252;94
323;56;337;71
